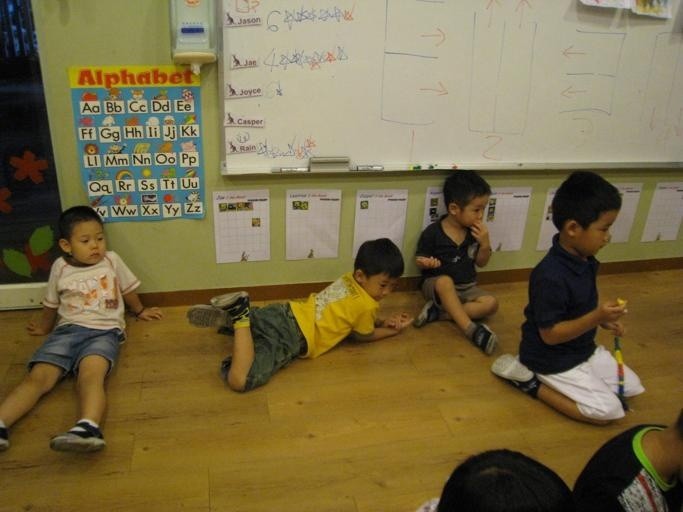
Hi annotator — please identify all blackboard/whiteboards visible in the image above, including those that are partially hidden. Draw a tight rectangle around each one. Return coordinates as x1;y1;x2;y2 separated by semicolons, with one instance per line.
218;0;683;176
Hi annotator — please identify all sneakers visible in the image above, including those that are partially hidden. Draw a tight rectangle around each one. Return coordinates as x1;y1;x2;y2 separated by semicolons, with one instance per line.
0;424;7;451
50;422;101;454
472;324;497;355
188;305;230;329
491;354;537;391
211;292;249;317
414;299;438;328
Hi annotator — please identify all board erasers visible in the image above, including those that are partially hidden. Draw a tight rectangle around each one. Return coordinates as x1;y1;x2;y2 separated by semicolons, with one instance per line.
308;157;352;174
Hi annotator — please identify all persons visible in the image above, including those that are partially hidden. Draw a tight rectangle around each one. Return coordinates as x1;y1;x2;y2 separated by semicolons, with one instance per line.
0;205;163;452
187;238;413;393
573;409;682;511
415;172;499;355
417;449;573;511
492;170;646;426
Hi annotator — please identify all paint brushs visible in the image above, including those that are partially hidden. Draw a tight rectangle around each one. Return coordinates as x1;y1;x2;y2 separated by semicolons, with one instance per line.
410;162;437;170
279;166;309;172
358;165;385;172
